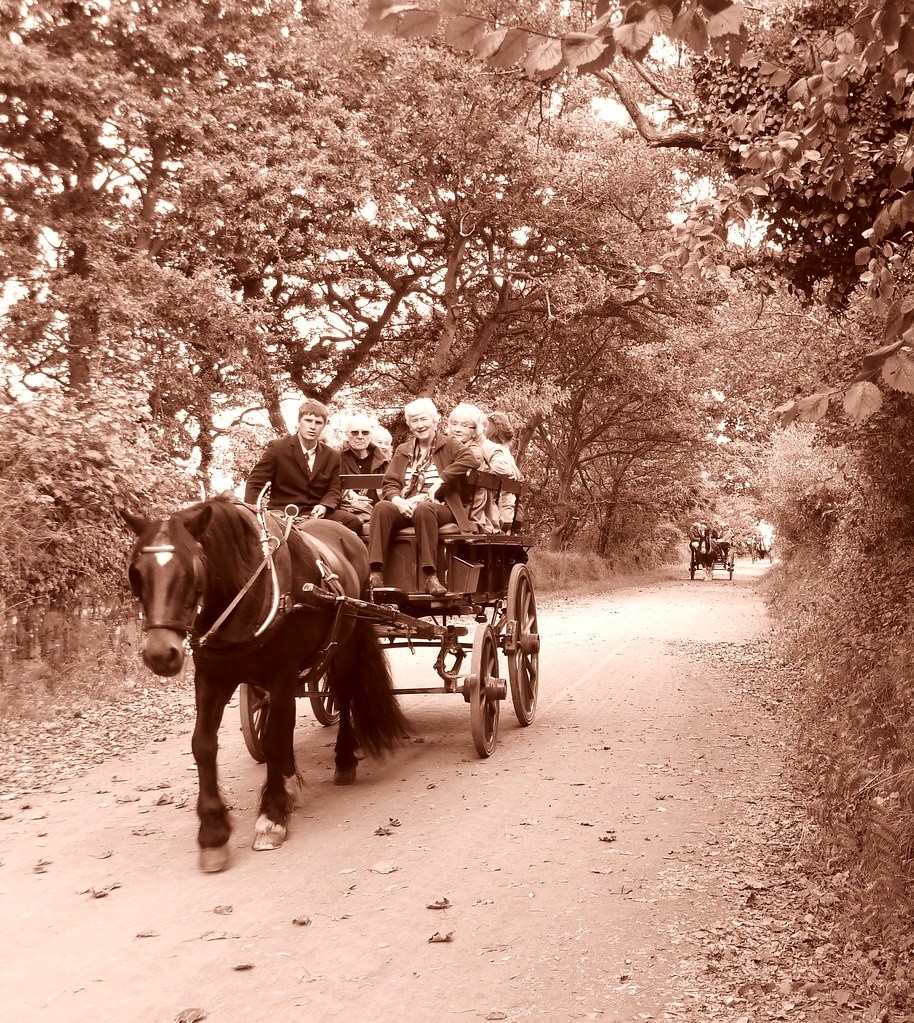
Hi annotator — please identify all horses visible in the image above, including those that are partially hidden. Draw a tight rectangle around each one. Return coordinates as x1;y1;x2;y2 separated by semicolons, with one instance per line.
117;492;419;873
695;526;718;581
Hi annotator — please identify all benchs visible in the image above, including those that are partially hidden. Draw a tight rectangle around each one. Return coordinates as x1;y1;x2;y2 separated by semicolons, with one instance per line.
338;473;536;592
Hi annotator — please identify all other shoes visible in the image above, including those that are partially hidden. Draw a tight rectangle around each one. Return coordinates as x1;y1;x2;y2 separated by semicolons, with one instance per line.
425;574;447;596
368;570;384;588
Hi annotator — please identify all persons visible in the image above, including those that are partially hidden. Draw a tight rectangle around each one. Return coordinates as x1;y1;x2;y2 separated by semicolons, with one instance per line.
689;518;733;542
338;401;526;535
244;399;363;535
367;396;481;596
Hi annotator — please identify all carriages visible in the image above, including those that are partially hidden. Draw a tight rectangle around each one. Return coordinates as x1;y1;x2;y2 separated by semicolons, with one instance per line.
751;536;773;564
117;468;541;873
689;526;735;583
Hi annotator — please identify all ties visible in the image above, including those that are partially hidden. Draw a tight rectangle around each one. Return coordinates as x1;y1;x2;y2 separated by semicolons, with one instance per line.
304;452;312;477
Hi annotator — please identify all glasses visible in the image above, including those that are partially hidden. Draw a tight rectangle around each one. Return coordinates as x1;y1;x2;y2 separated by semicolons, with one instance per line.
451;422;477;429
347;431;372;436
